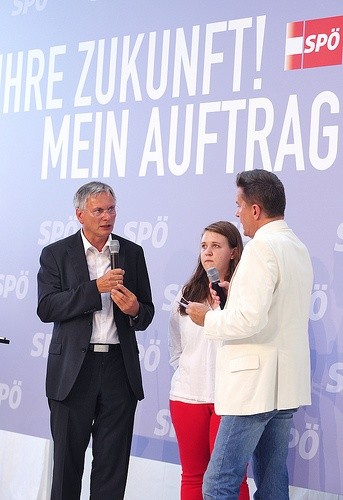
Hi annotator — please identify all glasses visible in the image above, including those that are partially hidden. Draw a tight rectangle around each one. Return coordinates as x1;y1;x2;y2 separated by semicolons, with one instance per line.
87;205;119;216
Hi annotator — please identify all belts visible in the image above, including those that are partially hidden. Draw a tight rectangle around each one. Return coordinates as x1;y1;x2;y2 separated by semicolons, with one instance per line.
88;343;121;353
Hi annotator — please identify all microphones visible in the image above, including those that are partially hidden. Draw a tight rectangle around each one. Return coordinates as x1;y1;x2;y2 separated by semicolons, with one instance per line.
109;240;120;270
207;267;227;310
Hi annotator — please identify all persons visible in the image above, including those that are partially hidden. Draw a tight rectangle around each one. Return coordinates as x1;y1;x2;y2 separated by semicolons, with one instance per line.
169;221;250;500
186;169;313;500
37;182;154;500
